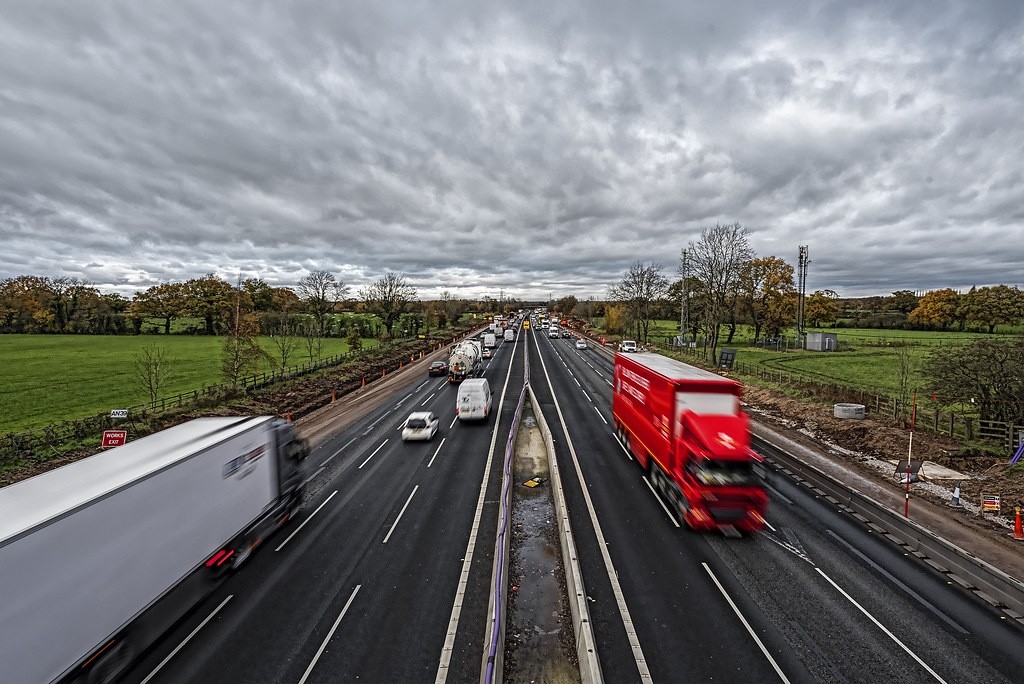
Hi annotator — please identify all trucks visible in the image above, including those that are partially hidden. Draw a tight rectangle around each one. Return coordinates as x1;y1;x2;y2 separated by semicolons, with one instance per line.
494;327;503;338
484;334;497;348
493;315;503;325
497;320;508;330
616;340;638;353
503;329;515;342
489;324;496;333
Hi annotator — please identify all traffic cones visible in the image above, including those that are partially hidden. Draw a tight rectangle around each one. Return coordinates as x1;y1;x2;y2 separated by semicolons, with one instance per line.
1013;506;1024;542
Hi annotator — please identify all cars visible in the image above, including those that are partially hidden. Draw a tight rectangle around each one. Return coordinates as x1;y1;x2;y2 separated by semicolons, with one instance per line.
428;361;449;377
482;348;491;359
503;308;525;332
575;339;588;351
480;331;487;339
531;306;571;339
401;411;439;442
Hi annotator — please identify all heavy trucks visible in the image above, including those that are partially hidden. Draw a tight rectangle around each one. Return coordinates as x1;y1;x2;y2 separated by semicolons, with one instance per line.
609;350;770;538
1;412;310;684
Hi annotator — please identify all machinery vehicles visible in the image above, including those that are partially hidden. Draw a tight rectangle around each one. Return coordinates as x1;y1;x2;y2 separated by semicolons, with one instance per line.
448;337;484;384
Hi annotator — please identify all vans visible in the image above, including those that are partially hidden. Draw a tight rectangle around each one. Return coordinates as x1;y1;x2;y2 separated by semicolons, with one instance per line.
455;378;495;422
548;326;560;338
